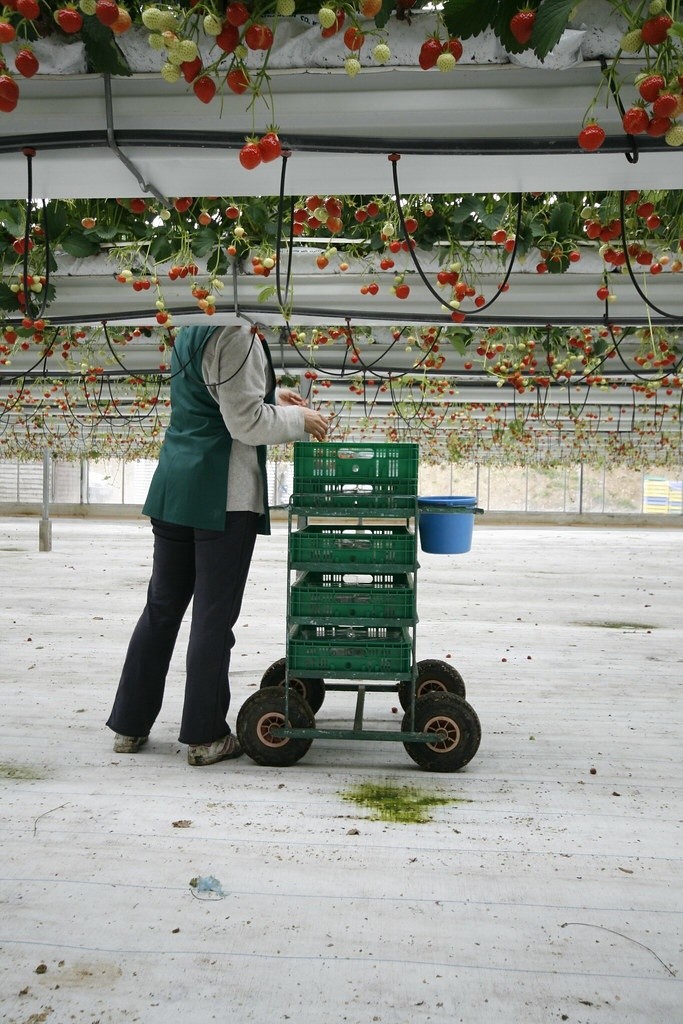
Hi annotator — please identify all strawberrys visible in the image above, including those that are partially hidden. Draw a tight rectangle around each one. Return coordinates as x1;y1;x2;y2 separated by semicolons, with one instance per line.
0;0;683;456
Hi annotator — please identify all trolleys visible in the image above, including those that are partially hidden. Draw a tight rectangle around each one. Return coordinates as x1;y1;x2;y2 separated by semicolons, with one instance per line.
236;439;481;774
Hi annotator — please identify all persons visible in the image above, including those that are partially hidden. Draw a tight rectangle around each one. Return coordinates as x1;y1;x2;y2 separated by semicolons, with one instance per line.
106;326;327;763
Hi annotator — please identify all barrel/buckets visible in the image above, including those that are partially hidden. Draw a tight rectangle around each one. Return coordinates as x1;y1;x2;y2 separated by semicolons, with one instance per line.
418;495;476;554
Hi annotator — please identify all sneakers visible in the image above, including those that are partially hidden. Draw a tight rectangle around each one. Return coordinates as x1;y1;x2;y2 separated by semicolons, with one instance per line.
187;732;243;764
114;733;149;753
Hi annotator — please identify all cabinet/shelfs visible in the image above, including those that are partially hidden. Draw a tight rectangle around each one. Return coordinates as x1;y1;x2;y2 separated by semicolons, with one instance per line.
236;492;482;775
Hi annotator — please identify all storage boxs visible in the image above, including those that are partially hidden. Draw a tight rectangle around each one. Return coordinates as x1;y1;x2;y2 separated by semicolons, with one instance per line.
290;441;420;674
